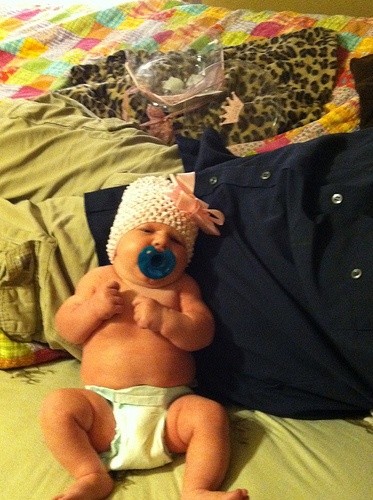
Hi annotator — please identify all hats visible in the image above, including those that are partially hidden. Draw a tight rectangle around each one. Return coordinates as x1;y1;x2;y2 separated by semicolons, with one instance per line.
106;172;225;264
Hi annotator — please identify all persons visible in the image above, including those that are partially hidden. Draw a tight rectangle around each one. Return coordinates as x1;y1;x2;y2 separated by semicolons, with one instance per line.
39;171;251;500
